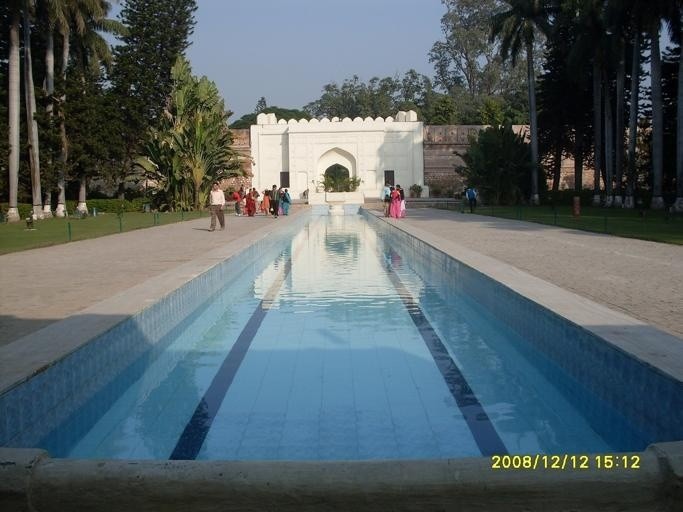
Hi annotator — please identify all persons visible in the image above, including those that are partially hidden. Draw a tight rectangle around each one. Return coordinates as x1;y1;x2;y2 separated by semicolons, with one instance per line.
465;188;478;212
230;185;290;215
381;180;406;217
208;182;226;230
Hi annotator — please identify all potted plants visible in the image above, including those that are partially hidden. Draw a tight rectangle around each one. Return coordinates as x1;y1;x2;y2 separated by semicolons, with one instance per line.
408;182;422;198
